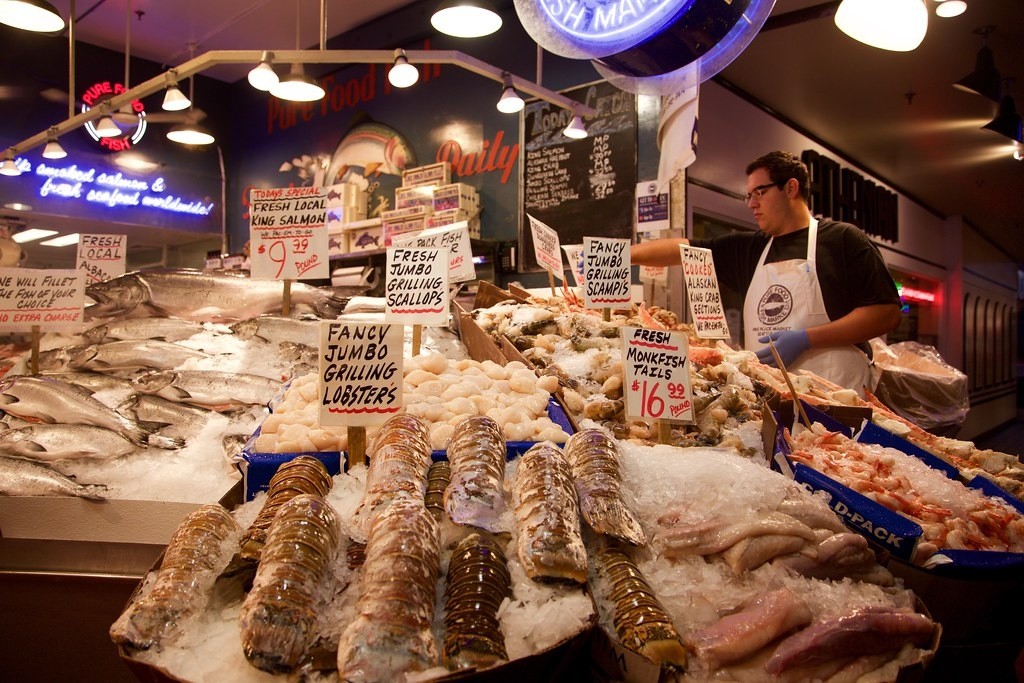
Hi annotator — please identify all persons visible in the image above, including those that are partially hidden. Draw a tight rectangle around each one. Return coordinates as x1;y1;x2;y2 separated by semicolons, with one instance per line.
579;148;900;402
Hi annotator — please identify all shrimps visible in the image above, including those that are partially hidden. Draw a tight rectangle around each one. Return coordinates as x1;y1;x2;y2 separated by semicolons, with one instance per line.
777;419;1024;568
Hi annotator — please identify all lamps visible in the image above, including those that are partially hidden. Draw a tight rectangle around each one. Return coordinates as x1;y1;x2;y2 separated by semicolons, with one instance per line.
96;103;122;136
496;75;525;114
166;123;216;145
980;97;1022;140
0;148;21;176
0;0;66;32
430;0;504;39
269;63;326;101
247;52;280;91
562;101;588;139
42;128;68;159
388;49;419;89
951;26;1004;103
160;69;191;111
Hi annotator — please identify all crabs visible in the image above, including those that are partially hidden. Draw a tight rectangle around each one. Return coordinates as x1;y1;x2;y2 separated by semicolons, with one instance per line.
744;362;1023;504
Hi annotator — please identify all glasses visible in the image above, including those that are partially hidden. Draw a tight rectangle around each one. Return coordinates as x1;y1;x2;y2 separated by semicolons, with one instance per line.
745;181;782;206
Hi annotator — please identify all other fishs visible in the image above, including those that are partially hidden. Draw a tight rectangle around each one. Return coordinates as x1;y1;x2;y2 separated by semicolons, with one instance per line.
1;270;356;504
474;297;765;468
